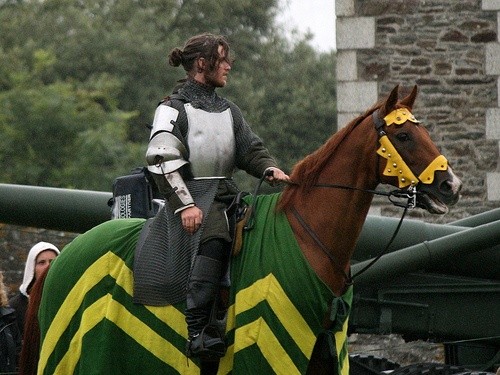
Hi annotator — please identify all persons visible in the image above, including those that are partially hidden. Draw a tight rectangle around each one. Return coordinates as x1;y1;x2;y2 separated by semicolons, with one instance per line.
0;270;22;375
133;33;291;358
7;242;61;342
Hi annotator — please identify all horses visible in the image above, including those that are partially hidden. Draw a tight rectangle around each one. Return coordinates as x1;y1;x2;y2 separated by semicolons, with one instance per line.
17;85;462;375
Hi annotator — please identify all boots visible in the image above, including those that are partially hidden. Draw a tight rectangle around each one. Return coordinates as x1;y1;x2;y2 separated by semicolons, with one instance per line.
185;255;226;358
